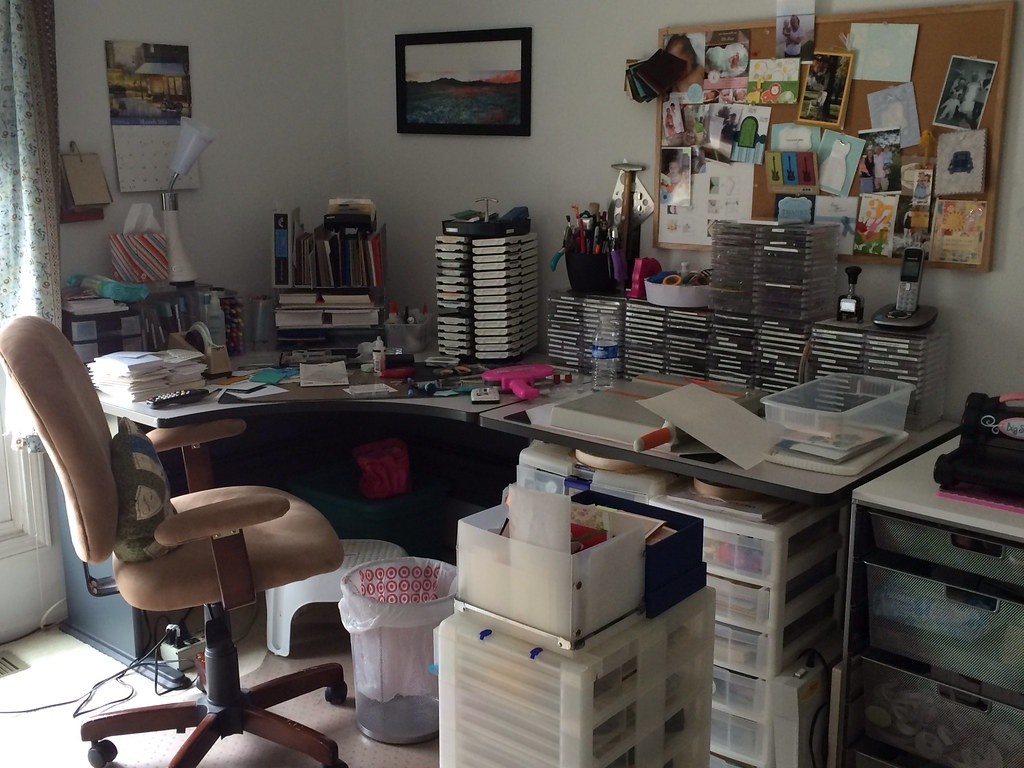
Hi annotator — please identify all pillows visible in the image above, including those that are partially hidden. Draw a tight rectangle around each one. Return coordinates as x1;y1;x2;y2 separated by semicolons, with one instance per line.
109;418;184;563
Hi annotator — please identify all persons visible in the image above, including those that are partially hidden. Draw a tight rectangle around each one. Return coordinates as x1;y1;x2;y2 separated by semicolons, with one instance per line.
939;69;993;121
720;113;738;143
665;103;698;147
783;15;804;58
666;159;681;184
665;34;704;92
693;146;706;173
859;144;895;193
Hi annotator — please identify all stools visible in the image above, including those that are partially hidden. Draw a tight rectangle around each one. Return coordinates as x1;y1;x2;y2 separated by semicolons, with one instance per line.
265;539;408;658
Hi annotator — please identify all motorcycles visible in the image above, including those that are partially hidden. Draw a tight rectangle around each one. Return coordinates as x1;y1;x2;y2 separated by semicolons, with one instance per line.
161;97;183;113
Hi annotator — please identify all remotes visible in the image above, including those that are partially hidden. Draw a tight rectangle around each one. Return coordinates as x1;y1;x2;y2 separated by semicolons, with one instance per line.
146;388;209;410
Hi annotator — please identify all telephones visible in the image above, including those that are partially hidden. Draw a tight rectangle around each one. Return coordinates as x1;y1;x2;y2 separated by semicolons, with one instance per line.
871;245;938;330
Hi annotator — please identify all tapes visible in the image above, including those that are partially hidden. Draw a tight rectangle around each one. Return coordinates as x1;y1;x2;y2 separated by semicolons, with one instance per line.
184;322;221;351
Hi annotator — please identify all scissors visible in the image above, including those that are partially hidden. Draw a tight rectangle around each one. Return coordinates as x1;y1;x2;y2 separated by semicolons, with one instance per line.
439;362;472;378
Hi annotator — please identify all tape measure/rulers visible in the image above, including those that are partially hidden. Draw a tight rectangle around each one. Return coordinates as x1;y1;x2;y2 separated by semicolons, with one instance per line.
611;159;654;242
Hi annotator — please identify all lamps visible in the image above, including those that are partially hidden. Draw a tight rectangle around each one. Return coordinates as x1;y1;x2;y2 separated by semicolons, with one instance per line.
161;116;213;283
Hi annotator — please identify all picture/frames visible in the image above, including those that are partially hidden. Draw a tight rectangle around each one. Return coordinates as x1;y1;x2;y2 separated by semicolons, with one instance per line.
395;26;532;137
646;0;1016;274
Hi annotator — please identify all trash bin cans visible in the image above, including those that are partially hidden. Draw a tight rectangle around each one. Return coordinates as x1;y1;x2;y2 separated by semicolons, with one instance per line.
337;556;459;745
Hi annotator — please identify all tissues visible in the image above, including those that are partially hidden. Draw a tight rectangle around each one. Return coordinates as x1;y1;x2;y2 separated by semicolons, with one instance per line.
109;201;167;284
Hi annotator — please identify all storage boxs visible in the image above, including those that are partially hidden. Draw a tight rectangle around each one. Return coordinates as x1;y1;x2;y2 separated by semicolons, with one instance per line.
762;371;916;452
382;314;433;353
435;444;836;768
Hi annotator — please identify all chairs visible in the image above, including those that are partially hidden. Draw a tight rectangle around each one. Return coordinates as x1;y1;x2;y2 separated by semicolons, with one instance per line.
2;317;347;768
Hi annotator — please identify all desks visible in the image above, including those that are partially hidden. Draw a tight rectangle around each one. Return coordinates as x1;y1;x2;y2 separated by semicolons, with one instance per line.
102;358;960;507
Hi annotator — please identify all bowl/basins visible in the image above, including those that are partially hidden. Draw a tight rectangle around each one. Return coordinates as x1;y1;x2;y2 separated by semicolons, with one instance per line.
643;272;710;308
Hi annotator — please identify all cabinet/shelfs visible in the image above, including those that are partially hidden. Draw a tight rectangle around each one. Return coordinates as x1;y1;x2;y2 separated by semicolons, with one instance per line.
837;434;1024;768
829;436;1024;768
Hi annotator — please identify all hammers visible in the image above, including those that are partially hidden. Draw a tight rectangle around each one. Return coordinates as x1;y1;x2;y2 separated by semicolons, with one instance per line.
611;163;645;253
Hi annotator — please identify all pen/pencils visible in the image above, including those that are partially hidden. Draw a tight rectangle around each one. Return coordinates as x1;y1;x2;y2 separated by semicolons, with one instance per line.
562;201;623;295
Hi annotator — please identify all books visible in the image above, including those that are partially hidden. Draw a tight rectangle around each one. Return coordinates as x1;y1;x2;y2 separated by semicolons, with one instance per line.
61;292;129;316
272;199;381;330
145;294;187;351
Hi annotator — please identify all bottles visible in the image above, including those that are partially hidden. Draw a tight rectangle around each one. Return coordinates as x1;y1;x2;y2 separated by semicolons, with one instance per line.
206;291;226;346
373;335;385;373
592;314;617;392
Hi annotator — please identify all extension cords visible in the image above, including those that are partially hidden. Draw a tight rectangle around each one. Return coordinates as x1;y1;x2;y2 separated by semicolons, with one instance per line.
160;632;206;672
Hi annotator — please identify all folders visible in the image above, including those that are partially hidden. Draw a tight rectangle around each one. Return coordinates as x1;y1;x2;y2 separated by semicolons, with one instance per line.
271;206;303;289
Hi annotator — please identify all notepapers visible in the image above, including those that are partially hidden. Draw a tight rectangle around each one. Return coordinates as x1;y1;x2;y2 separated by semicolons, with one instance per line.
249;369;286;385
